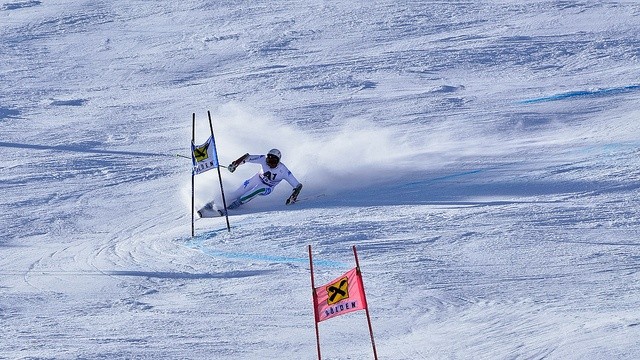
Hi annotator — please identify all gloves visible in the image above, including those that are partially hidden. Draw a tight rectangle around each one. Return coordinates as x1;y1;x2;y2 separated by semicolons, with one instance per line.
228;161;238;172
286;194;296;204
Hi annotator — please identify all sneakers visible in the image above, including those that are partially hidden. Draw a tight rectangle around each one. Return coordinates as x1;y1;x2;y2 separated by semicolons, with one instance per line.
217;209;226;216
204;202;213;209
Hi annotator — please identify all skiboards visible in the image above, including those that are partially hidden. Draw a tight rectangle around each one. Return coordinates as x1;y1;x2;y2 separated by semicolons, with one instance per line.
197;207;225;218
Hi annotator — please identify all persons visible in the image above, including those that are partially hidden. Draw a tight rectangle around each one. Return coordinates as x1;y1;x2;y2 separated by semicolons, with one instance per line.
203;149;301;216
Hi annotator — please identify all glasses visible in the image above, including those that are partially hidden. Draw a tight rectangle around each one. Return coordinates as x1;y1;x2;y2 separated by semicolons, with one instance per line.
266;158;278;164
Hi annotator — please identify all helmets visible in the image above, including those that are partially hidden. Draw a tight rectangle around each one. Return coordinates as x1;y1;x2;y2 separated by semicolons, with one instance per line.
267;149;281;164
